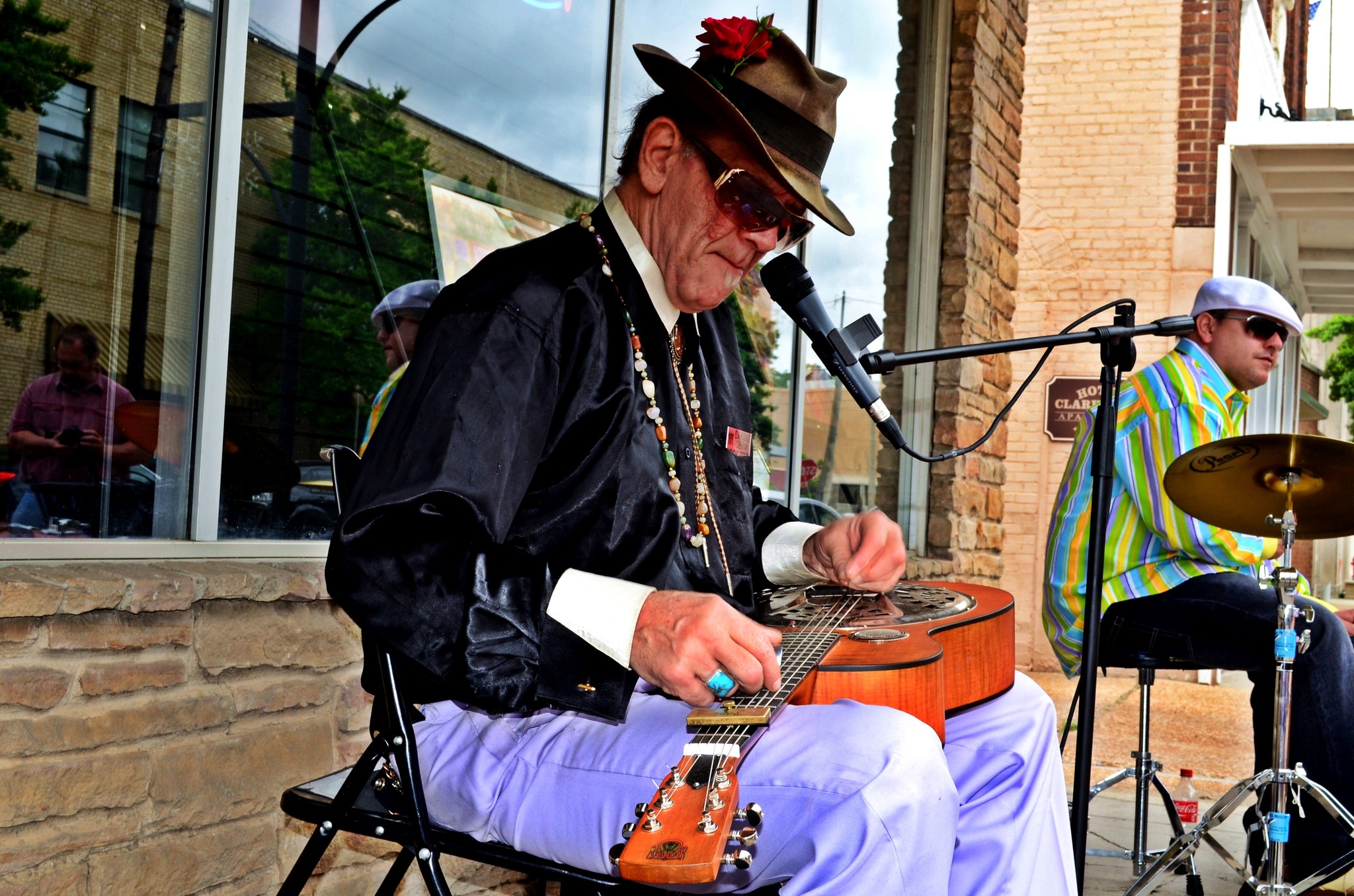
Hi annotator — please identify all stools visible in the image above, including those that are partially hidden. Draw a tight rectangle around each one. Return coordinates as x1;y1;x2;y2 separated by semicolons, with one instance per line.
1089;651;1218;896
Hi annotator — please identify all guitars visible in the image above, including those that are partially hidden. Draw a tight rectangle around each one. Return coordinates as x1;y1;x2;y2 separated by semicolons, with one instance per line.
607;579;1017;886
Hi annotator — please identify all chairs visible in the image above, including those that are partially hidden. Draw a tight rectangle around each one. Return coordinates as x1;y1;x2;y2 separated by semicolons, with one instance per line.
278;446;621;896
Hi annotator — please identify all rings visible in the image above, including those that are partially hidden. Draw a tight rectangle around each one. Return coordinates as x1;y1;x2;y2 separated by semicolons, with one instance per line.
706;669;734;698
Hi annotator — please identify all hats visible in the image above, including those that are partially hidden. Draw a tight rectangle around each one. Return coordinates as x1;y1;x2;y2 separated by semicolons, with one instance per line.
1192;275;1303;337
631;13;856;236
372;279;444;320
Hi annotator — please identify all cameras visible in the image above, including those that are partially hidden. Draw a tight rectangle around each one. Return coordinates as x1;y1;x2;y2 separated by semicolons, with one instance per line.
58;426;84;446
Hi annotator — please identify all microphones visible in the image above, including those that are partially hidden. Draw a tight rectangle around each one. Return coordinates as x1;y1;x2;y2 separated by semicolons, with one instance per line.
759;252;908;449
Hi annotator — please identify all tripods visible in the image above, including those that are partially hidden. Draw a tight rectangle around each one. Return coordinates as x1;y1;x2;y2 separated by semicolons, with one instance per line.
1125;476;1354;896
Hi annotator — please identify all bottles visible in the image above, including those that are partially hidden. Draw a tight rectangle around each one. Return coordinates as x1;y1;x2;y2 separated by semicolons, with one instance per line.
1169;768;1199;858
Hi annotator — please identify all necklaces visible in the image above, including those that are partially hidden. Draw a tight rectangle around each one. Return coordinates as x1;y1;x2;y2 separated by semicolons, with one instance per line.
575;208;737;601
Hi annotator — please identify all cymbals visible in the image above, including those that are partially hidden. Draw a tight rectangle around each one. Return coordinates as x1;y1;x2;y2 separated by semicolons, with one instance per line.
1163;433;1354;541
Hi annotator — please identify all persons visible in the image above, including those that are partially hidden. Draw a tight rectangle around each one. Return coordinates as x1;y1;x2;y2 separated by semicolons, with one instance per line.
326;21;1082;896
358;278;442;458
1040;275;1354;896
7;323;141;539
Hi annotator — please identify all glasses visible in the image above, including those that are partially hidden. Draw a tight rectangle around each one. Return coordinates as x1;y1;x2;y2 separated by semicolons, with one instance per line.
1222;315;1289;352
56;358;84;371
382;314;418;334
683;128;818;255
